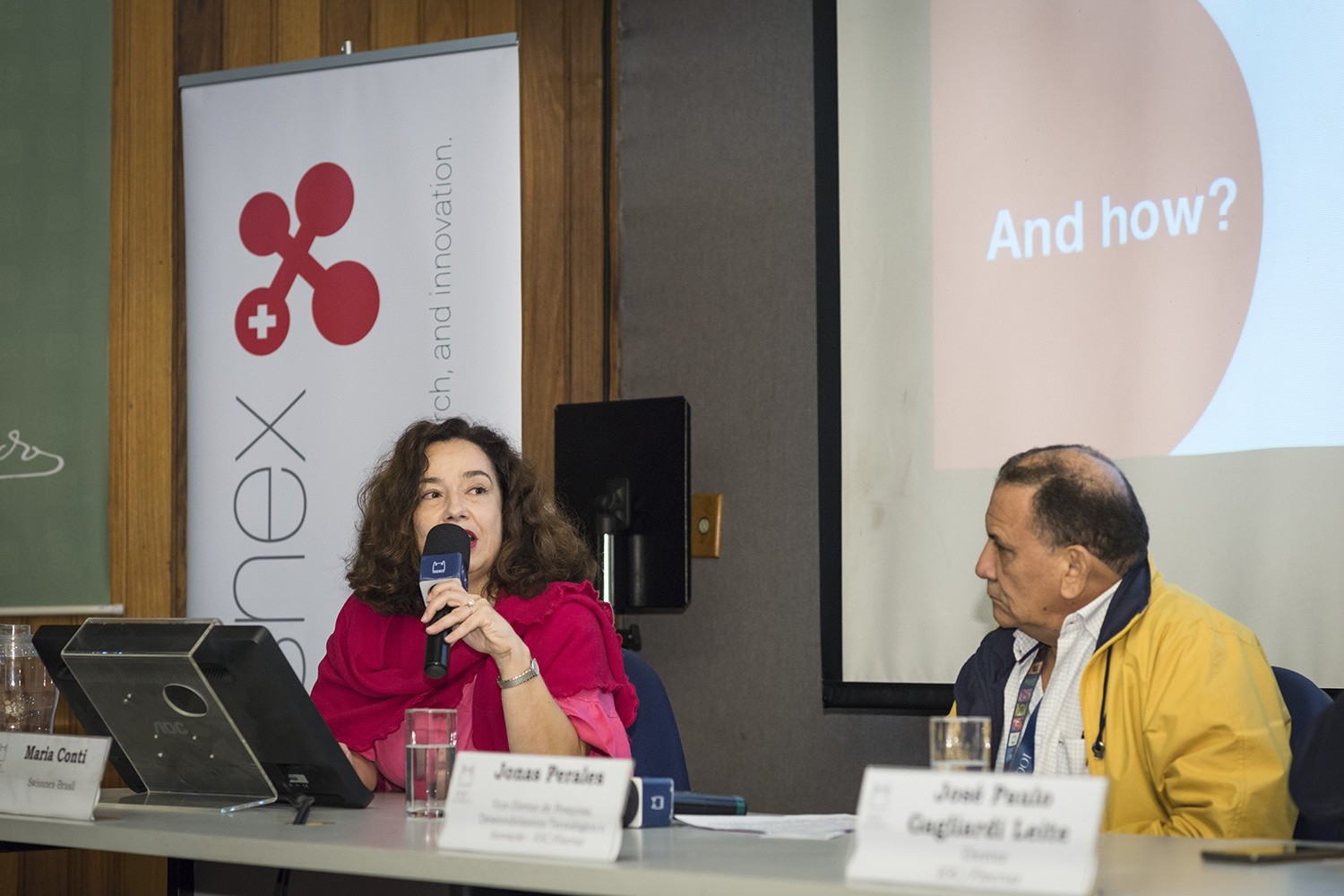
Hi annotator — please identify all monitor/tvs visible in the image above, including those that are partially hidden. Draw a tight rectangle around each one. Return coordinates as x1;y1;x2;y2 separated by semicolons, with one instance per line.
32;624;376;809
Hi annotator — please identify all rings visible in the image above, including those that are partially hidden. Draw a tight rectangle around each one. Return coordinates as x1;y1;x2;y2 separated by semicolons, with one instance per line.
461;600;474;614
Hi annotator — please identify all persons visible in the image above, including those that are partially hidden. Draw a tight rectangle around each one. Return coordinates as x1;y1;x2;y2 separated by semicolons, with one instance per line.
939;446;1299;864
308;418;633;803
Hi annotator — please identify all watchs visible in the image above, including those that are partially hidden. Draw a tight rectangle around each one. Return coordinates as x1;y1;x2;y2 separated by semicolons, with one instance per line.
498;657;541;689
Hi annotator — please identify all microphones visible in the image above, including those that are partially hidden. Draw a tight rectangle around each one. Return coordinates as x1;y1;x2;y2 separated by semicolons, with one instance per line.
418;524;470;679
622;777;749;830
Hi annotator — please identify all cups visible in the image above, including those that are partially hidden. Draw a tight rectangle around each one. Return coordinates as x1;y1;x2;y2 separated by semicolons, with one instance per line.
406;709;457;818
928;716;990;772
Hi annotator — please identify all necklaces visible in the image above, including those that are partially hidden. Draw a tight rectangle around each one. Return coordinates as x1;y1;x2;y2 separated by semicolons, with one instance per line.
1002;645;1048;776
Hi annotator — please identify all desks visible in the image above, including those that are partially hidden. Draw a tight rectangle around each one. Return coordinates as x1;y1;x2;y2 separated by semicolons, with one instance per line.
0;789;1344;896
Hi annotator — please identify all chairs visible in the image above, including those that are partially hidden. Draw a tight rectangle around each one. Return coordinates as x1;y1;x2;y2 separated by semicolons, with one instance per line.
1270;664;1344;841
619;649;693;791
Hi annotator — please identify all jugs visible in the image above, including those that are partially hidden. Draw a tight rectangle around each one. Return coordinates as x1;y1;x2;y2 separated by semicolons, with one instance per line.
0;623;60;735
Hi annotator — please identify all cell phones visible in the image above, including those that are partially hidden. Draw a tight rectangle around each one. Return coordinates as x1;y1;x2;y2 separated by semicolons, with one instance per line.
1200;843;1344;864
